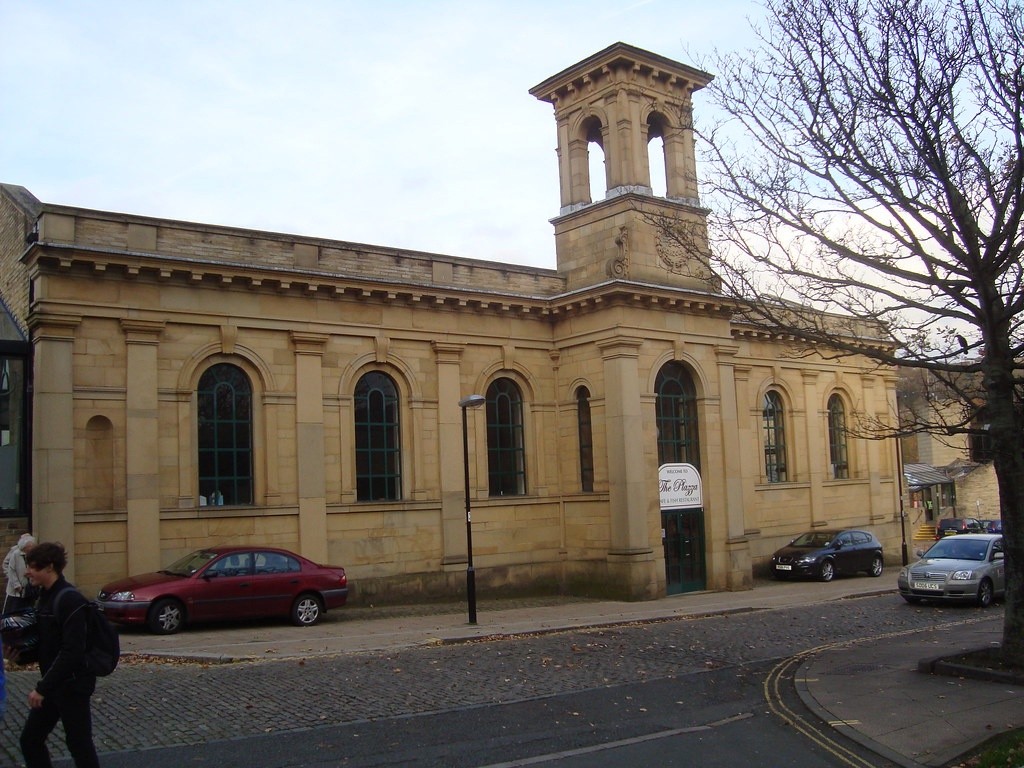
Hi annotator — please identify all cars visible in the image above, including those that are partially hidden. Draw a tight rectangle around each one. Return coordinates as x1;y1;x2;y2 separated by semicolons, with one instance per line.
769;529;884;582
92;543;350;634
897;534;1006;608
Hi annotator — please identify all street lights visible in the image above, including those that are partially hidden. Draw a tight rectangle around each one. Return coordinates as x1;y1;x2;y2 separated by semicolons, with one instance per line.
458;394;488;625
893;429;916;566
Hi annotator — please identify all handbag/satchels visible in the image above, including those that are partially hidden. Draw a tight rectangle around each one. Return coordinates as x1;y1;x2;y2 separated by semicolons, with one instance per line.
24;583;36;604
4;591;33;618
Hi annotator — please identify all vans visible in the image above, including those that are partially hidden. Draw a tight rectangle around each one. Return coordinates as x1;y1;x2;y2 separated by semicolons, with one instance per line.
934;517;1003;541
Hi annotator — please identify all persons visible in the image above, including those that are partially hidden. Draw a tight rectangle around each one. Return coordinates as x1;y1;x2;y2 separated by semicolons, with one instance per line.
926;498;933;520
2;533;38;665
2;541;101;768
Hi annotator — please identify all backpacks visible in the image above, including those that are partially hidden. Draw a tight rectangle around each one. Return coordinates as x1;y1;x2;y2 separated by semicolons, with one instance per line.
34;588;120;677
